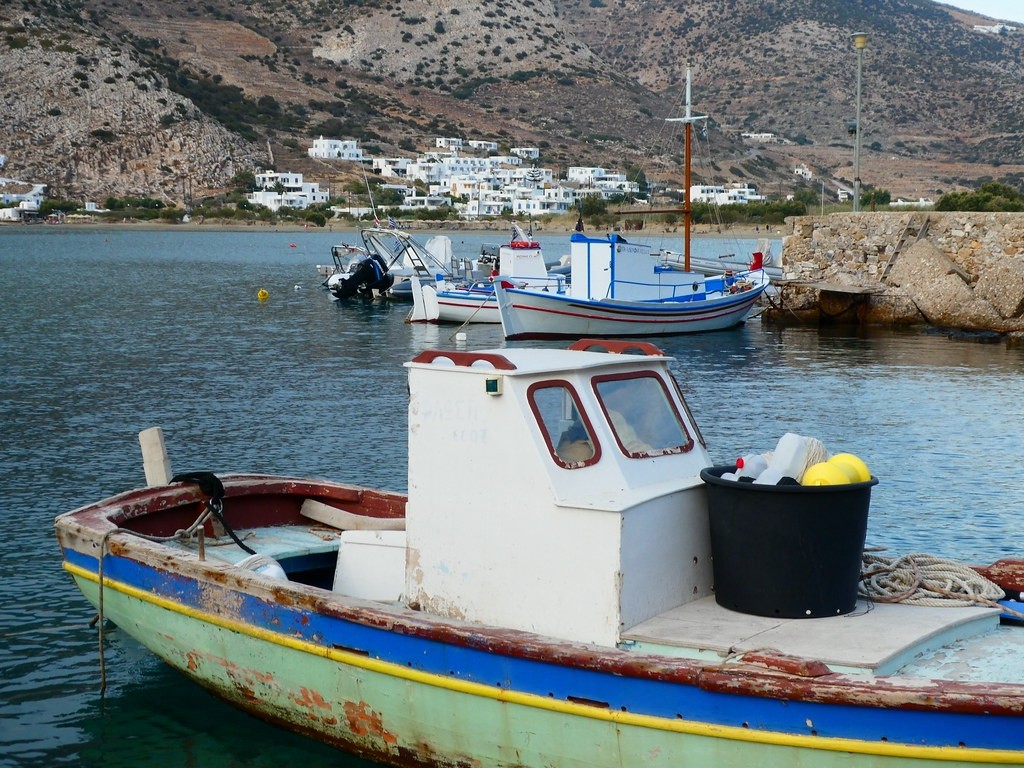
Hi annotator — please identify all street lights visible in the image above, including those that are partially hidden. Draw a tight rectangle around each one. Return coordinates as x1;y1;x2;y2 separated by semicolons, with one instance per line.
851;30;872;212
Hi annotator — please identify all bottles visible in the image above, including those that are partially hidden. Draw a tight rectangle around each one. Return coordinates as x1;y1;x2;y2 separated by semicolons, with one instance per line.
718;433;871;485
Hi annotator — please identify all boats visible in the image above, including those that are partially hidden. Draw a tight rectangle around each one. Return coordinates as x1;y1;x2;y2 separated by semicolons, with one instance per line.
322;153;571;326
53;323;1023;768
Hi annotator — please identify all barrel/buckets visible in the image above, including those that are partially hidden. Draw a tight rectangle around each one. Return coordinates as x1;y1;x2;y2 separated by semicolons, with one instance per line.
700;465;879;619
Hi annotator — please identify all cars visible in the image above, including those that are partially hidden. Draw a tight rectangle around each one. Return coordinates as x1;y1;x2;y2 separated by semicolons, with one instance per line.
665;198;679;206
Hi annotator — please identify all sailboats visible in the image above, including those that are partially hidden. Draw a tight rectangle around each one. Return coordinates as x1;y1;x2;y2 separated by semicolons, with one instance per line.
487;66;771;341
